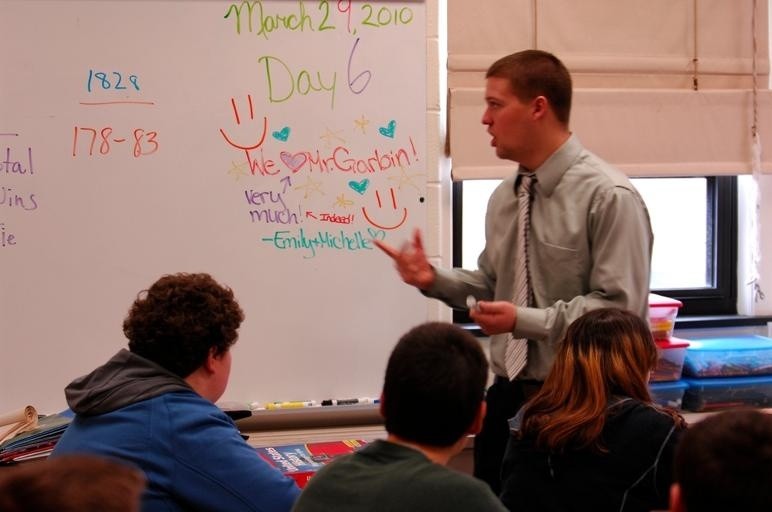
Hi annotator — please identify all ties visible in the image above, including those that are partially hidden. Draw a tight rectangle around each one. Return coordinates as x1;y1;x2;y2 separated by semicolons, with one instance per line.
501;174;535;382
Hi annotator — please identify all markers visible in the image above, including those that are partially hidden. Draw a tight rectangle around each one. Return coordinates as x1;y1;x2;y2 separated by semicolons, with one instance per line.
265;399;380;410
466;295;481;312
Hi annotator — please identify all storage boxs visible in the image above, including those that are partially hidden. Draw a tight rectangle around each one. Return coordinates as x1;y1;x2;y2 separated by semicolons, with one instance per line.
646;292;772;414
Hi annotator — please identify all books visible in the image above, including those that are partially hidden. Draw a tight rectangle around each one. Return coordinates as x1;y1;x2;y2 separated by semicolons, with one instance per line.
1;404;76;465
247;438;371;492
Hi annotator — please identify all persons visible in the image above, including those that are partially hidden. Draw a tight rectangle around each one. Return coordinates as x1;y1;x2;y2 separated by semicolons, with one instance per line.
370;49;653;495
493;305;690;511
290;321;507;511
661;406;772;511
44;272;305;511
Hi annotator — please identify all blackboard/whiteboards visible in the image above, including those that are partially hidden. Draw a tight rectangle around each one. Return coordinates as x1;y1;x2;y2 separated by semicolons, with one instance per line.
0;0;455;431
